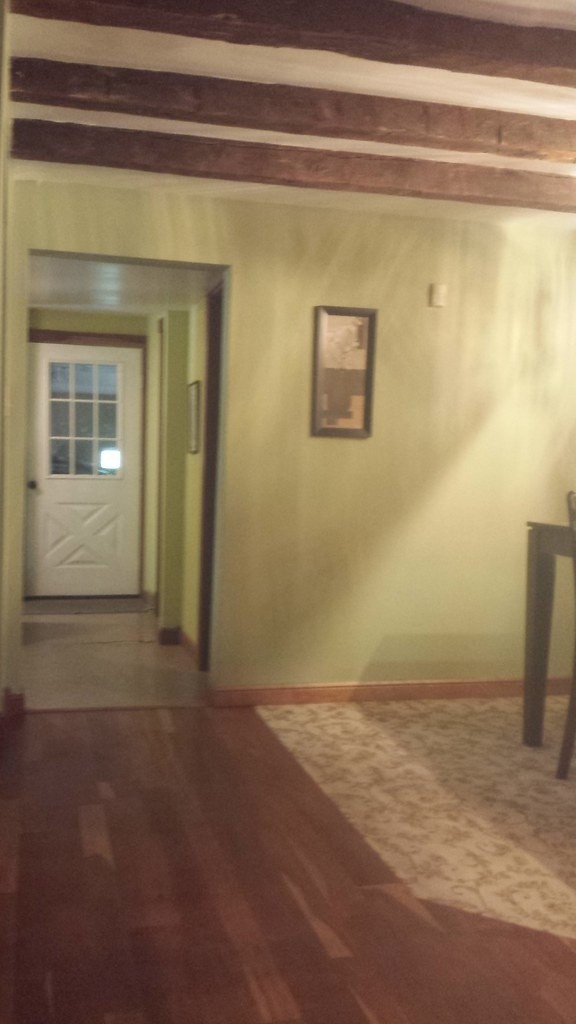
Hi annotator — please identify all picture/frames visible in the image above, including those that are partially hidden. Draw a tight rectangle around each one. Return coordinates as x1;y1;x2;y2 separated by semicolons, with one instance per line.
311;306;378;439
184;380;201;452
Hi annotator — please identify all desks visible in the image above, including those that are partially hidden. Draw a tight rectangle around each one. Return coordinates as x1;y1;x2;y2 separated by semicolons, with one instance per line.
524;520;576;777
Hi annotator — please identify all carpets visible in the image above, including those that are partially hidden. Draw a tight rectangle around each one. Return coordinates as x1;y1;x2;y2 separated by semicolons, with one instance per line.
255;692;576;938
22;596;154;616
